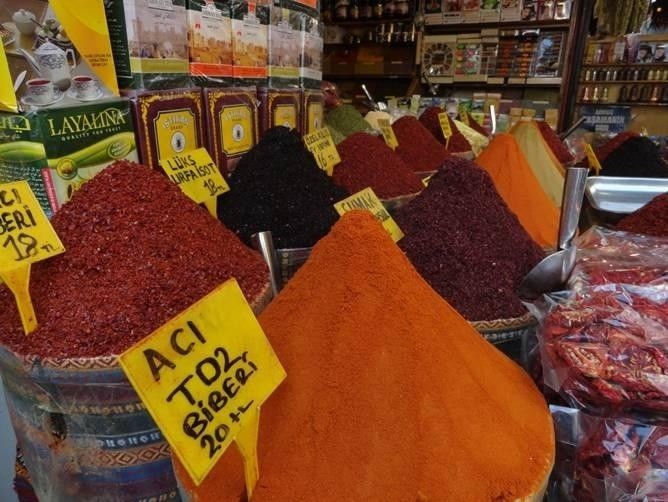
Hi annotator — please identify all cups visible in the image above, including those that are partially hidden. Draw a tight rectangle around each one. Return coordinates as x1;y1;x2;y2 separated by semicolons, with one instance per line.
12;9;36;35
26;78;59;103
72;75;101;97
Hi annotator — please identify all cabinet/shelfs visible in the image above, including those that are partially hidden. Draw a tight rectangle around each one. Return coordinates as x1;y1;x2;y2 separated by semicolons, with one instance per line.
565;0;668;138
318;1;579;134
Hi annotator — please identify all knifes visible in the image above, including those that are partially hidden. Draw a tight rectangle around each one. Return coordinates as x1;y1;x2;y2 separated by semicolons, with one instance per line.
15;71;27;92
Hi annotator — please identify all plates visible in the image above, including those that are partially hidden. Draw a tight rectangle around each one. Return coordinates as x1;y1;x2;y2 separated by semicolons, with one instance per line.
21;91;64;106
38;33;75;59
68;87;103;101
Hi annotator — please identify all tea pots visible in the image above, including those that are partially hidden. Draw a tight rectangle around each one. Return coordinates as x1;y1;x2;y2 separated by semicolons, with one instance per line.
20;38;77;89
